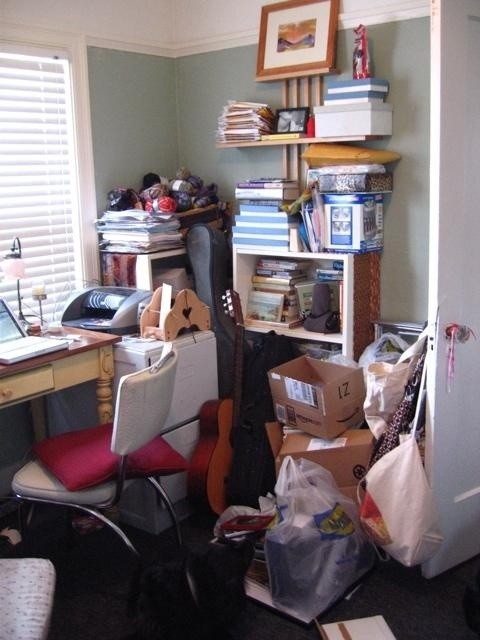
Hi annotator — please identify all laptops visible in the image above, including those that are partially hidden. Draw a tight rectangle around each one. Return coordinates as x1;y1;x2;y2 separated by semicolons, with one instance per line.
0;297;69;365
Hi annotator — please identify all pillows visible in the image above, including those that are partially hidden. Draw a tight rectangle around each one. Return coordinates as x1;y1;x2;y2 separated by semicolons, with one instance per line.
25;421;191;493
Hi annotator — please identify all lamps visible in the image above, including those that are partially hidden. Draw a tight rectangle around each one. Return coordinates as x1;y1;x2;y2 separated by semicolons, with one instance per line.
2;236;48;335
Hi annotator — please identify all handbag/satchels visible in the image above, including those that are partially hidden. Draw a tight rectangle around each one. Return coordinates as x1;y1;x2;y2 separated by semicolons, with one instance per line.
357;392;444;568
132;530;265;639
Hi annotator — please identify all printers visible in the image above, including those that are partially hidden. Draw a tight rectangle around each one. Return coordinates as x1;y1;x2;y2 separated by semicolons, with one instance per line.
61;285;154;335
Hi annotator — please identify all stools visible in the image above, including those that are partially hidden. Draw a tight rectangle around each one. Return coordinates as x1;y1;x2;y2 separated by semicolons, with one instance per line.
0;556;56;640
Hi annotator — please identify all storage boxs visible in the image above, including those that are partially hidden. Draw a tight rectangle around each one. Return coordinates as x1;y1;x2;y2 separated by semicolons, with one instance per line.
264;352;376;507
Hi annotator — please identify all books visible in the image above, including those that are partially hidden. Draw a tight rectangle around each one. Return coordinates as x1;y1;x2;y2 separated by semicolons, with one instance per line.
94;209;185;255
242;254;345;335
246;178;295;183
238;183;299;188
260;133;307;139
241;200;295;205
320;613;397;640
235;187;300;200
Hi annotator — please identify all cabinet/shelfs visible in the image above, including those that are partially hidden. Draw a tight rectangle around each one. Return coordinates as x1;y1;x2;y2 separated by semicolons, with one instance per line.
100;246;195;292
0;326;124;527
45;326;221;537
215;65;384;365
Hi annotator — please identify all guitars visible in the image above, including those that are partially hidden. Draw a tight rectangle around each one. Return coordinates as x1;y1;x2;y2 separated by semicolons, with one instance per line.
185;288;244;525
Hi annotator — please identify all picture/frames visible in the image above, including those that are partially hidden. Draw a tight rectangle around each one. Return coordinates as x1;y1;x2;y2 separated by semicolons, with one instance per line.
255;0;339;77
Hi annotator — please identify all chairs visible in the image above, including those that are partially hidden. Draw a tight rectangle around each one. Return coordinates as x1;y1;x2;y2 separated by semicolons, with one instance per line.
11;340;209;622
